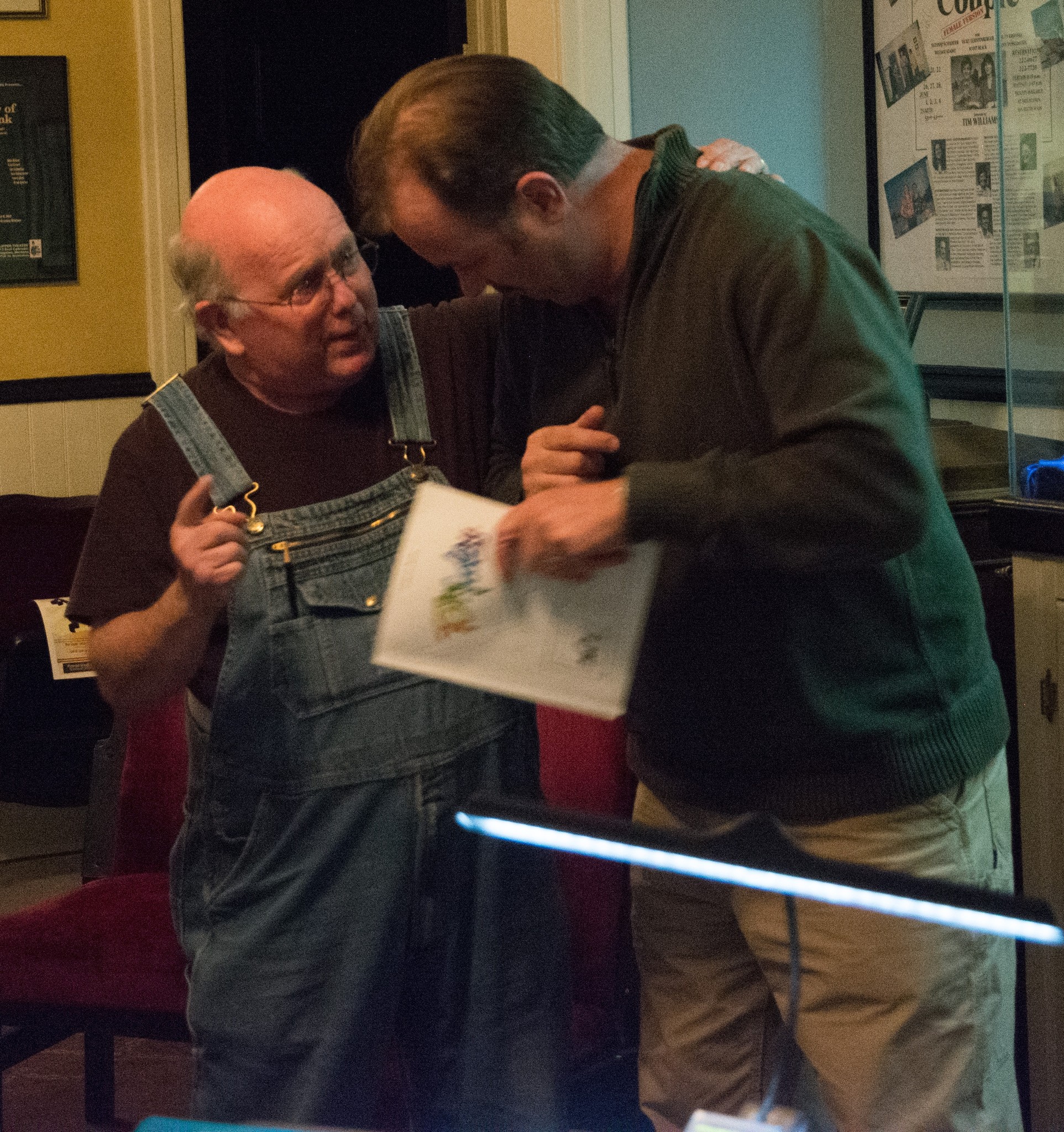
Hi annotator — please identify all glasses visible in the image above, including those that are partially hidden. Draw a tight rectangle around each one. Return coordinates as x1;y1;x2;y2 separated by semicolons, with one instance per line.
222;236;380;317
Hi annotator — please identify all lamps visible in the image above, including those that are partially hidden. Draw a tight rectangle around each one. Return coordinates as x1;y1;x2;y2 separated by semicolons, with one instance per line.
450;794;1064;1132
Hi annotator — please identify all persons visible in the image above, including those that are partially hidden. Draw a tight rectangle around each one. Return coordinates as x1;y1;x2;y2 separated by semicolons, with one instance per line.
954;58;981;110
354;54;1023;1132
1024;236;1040;269
899;182;924;226
885;51;915;97
935;239;950;270
976;170;991;196
933;143;945;174
1021;140;1036;168
979;54;1007;108
978;209;992;240
65;138;784;1132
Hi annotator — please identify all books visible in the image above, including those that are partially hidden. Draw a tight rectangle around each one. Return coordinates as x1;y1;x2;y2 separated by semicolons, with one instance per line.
367;480;663;723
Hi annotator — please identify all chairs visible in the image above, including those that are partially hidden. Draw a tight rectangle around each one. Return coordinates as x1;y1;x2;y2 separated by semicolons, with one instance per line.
0;491;637;1132
